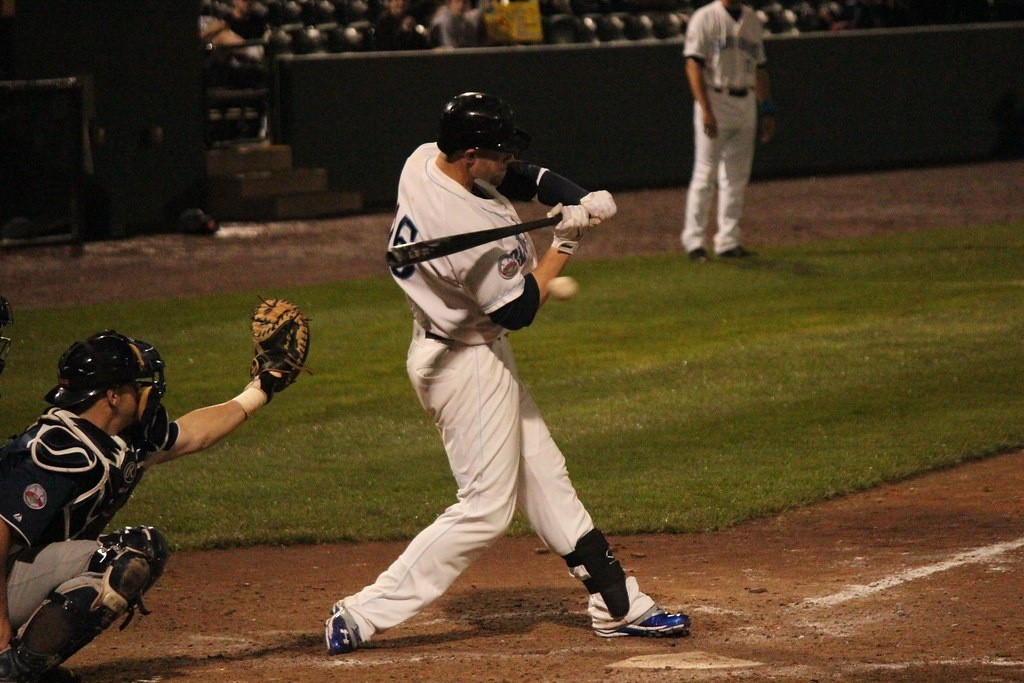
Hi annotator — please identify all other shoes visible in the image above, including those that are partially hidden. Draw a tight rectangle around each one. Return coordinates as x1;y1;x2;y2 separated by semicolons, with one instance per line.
689;247;710;263
722;245;758;259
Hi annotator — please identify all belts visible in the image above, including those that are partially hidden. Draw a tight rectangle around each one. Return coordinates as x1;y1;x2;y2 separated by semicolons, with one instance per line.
713;87;749;97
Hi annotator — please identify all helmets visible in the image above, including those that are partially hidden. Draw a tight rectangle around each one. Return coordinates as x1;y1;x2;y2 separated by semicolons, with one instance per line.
437;91;535;154
44;330;162;410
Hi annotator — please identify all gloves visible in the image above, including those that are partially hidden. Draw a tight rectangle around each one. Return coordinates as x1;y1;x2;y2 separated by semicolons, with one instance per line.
546;189;618;254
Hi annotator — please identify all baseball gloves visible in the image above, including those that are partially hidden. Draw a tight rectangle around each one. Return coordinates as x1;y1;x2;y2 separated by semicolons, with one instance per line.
249;299;311;393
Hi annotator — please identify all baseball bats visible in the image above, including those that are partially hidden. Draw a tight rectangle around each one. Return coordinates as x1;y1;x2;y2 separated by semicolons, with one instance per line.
385;213;592;269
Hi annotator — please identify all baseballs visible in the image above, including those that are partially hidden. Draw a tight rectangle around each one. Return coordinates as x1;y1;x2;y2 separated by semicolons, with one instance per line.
546;276;578;301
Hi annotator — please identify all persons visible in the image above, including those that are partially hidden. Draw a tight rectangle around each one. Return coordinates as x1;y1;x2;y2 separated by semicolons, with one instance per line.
224;0;477;55
325;92;691;657
0;296;311;683
669;0;777;261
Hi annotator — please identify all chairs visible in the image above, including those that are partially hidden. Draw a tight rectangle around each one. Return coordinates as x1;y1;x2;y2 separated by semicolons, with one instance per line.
196;0;1024;125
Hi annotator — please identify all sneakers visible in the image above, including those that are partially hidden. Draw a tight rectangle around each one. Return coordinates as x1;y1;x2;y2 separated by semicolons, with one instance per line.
594;604;691;638
325;599;363;656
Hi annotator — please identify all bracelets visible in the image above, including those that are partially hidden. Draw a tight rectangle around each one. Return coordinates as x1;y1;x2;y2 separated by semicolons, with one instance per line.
234;389;268;418
758;101;774;116
